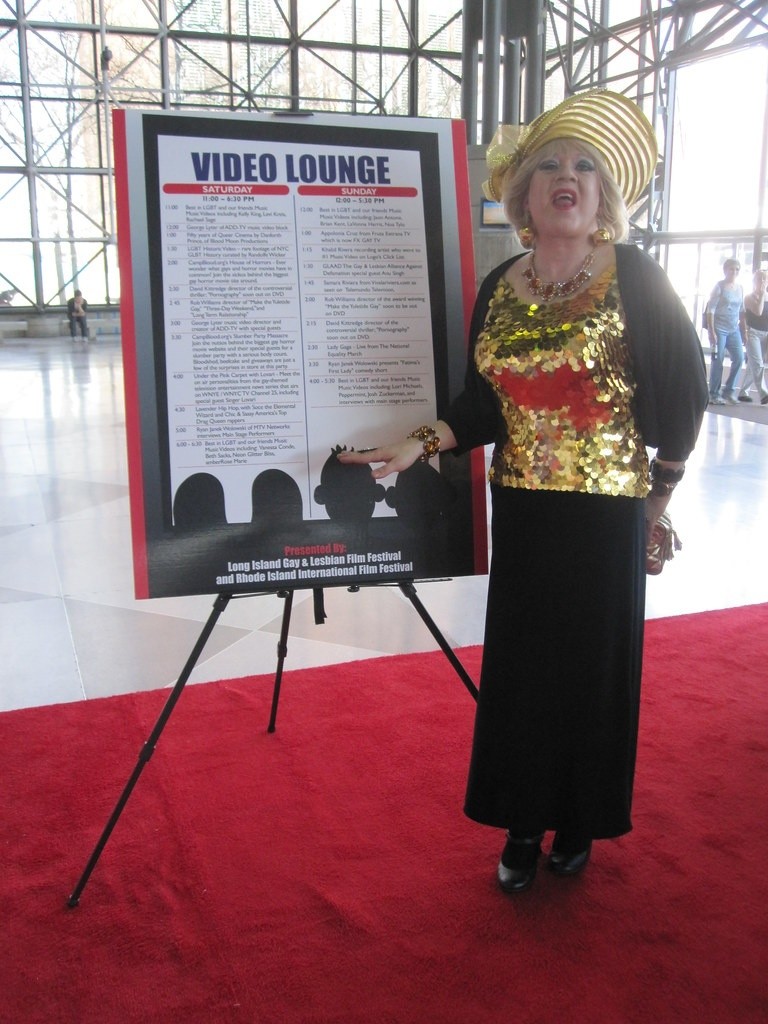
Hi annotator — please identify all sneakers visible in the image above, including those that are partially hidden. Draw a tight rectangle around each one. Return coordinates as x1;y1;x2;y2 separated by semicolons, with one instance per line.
723;394;740;406
709;395;725;404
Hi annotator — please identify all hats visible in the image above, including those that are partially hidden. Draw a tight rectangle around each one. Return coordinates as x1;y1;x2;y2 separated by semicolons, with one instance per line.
483;90;656;209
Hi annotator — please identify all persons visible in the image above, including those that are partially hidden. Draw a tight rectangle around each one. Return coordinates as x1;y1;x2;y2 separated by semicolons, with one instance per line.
337;90;708;893
67;290;89;341
738;271;768;405
706;259;748;404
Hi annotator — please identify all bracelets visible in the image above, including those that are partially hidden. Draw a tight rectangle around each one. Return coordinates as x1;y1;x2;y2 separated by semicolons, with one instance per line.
648;457;686;482
407;426;441;460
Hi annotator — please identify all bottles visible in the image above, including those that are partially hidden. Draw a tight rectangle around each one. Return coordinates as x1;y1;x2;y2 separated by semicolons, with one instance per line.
711;344;718;360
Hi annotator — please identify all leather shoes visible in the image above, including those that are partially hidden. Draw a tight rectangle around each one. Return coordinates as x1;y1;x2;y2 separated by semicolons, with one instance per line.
499;830;544;891
545;846;588;875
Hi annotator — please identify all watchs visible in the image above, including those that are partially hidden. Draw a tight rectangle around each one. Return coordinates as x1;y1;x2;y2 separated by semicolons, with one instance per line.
649;475;678;496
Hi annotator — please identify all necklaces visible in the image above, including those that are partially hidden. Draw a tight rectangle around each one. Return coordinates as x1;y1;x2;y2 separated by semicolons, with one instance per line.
522;242;599;301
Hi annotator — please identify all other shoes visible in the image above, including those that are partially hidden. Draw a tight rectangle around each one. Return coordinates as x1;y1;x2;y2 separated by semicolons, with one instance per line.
761;395;768;405
737;396;752;403
